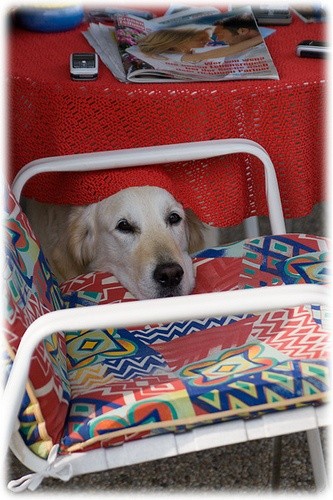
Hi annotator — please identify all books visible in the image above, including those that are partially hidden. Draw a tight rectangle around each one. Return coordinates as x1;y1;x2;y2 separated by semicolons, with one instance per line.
110;4;279;83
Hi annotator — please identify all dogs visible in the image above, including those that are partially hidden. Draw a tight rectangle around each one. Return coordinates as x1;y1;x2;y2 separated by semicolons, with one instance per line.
19;186;211;302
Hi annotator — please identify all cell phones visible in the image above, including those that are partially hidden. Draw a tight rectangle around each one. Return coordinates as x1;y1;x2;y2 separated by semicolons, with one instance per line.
295;38;330;60
69;51;99;81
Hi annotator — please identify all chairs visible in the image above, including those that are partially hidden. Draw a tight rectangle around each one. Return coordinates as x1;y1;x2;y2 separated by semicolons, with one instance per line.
6;138;328;491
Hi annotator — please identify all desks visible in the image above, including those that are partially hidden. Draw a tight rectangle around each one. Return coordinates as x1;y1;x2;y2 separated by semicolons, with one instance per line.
7;10;327;240
